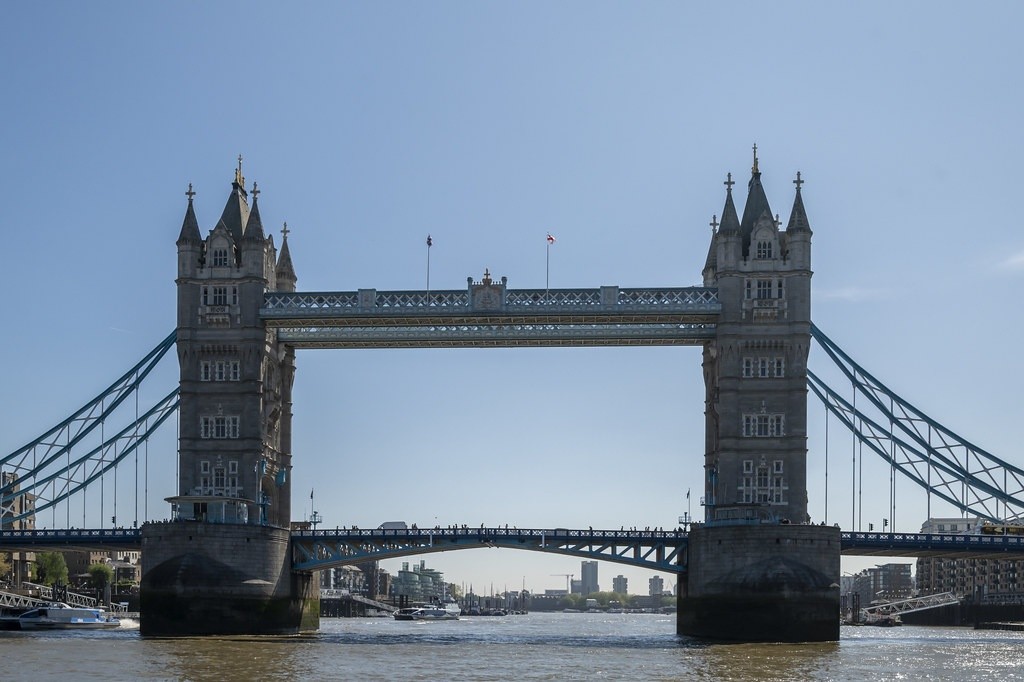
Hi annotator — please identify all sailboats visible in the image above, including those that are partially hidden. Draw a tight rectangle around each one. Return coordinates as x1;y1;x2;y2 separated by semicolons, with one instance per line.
462;576;530;616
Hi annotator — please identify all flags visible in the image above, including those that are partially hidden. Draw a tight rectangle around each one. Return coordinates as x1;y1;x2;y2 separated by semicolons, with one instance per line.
547;234;555;244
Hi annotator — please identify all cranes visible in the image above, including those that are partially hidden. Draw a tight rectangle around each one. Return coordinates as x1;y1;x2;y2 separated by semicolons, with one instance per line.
551;574;575;594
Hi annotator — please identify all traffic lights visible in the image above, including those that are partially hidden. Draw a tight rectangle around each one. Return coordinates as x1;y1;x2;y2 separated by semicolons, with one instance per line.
871;524;874;531
134;521;137;528
112;517;114;523
885;519;889;526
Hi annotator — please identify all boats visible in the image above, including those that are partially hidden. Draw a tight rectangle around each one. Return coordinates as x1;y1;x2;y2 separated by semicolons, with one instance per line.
0;602;121;630
392;606;460;621
